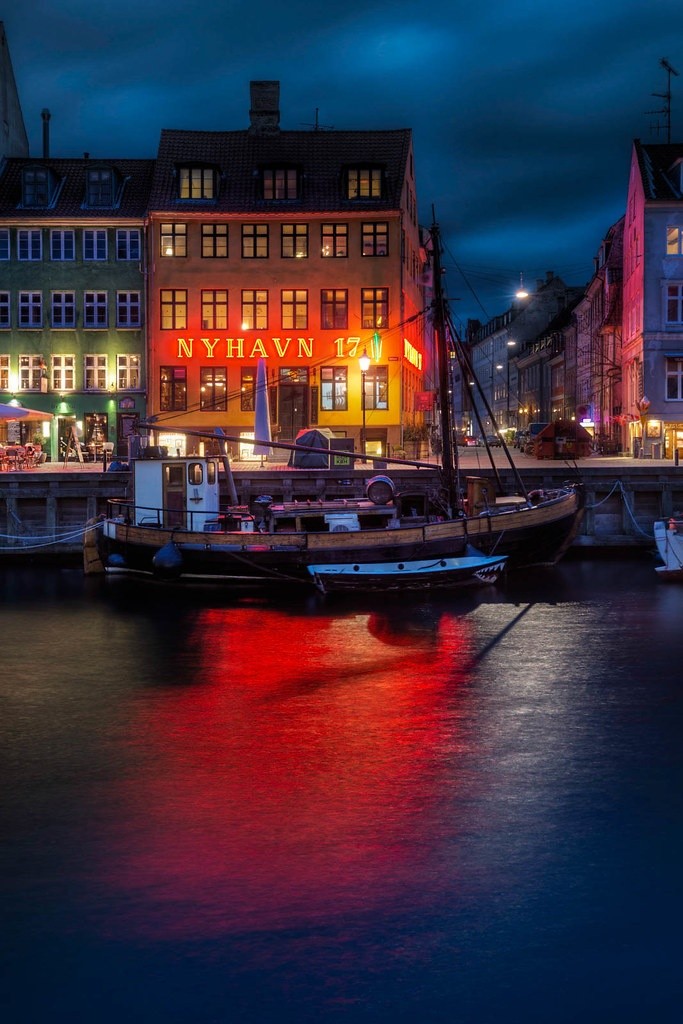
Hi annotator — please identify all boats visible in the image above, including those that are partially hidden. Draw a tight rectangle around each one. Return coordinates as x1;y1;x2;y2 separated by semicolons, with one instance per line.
1;516;123;556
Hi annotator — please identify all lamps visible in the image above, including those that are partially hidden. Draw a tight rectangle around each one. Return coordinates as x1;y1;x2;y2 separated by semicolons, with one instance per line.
58;398;69;414
108;396;115;408
10;396;20;407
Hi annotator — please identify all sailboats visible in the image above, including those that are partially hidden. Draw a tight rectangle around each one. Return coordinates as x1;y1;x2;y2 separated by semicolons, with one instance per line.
99;203;582;602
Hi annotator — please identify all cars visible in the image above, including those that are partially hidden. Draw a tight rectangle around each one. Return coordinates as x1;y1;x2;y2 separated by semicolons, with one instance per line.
514;430;530;453
486;436;501;447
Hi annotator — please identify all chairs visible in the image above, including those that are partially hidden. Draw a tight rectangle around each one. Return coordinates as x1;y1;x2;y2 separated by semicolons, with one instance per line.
0;445;42;472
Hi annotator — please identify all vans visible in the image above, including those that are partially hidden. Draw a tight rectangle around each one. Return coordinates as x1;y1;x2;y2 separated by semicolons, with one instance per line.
527;422;550;443
